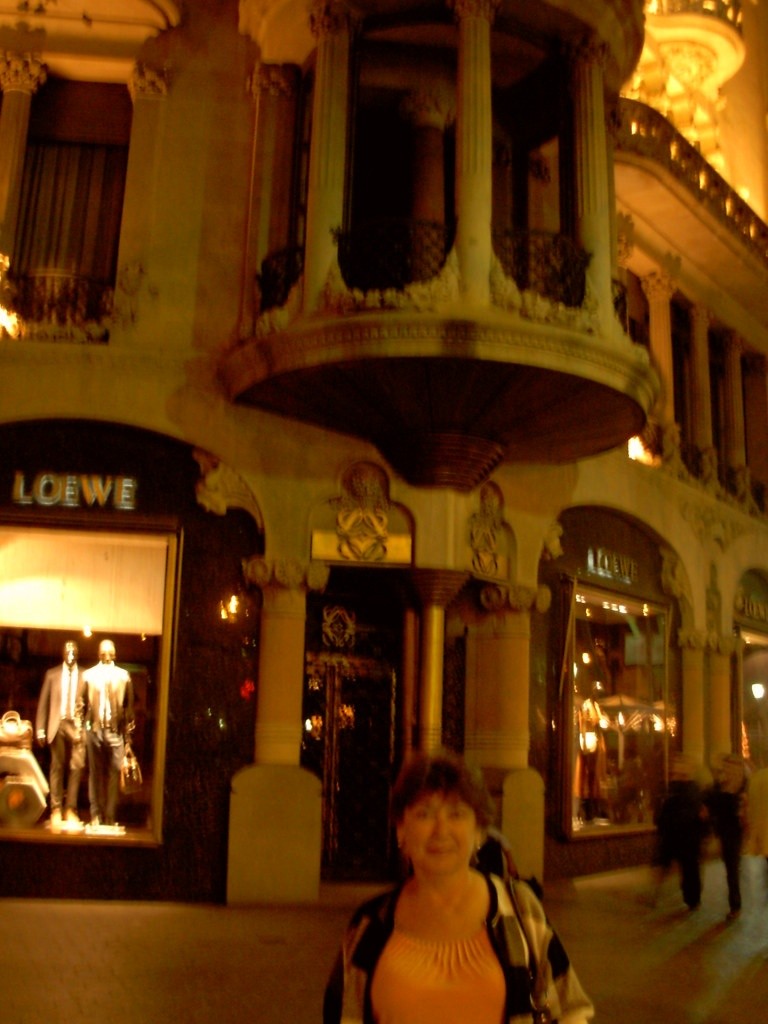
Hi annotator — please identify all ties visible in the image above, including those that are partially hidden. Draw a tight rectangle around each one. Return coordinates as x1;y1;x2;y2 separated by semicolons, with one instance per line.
65;667;73;719
103;675;107;727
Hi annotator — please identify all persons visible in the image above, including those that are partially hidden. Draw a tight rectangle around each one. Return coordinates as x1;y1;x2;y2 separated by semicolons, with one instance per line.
640;749;768;918
73;640;136;828
37;639;88;827
322;751;594;1024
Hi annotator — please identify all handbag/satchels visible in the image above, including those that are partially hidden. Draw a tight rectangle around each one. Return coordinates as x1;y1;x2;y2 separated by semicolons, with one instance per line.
120;743;142;794
0;710;33;745
